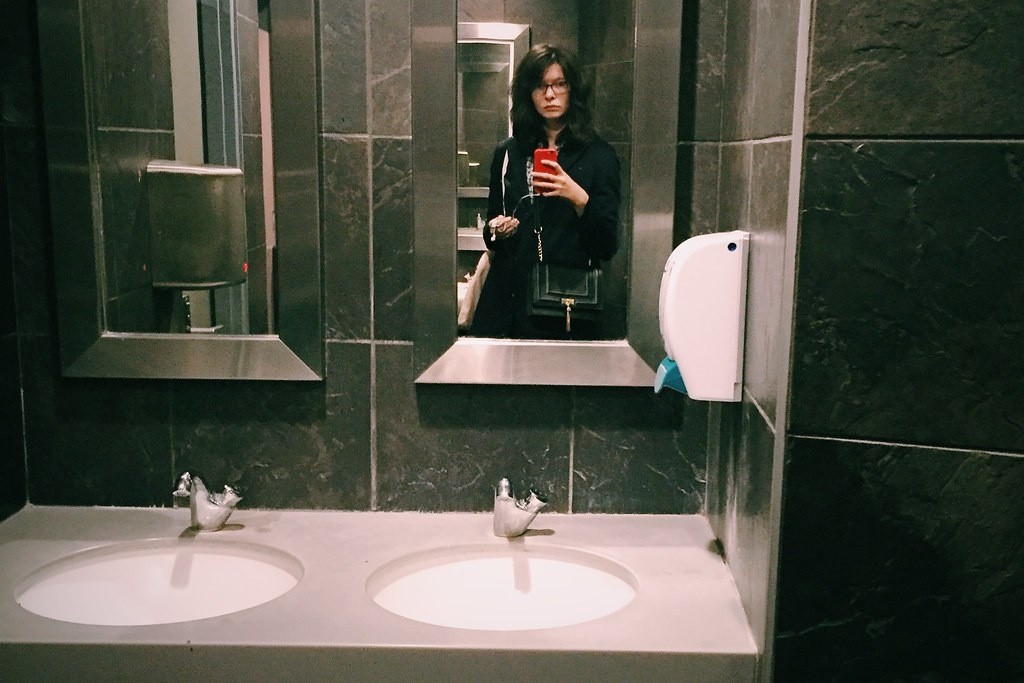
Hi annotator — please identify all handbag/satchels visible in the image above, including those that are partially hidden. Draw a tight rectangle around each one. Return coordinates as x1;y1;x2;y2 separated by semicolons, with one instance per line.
527;260;605;332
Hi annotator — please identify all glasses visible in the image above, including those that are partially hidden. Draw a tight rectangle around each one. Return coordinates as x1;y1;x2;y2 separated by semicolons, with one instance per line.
538;81;568;95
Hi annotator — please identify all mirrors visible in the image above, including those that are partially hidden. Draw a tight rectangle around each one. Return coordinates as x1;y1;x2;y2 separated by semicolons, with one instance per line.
86;0;267;336
456;4;635;345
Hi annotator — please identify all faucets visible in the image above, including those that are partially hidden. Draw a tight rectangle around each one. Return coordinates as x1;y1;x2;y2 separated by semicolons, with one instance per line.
485;460;546;538
475;213;487;229
171;472;241;533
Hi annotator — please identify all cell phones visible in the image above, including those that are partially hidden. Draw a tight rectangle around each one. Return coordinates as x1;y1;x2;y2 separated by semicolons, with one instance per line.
533;149;558;194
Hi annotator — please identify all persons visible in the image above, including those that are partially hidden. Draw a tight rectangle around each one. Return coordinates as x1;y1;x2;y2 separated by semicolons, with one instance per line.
468;44;621;341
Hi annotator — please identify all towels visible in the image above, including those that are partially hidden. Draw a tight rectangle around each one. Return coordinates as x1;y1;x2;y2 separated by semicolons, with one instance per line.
457;253;490;326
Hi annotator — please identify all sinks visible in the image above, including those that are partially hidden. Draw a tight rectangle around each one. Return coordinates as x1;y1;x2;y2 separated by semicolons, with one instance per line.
13;538;304;626
366;542;642;629
457;227;489;250
